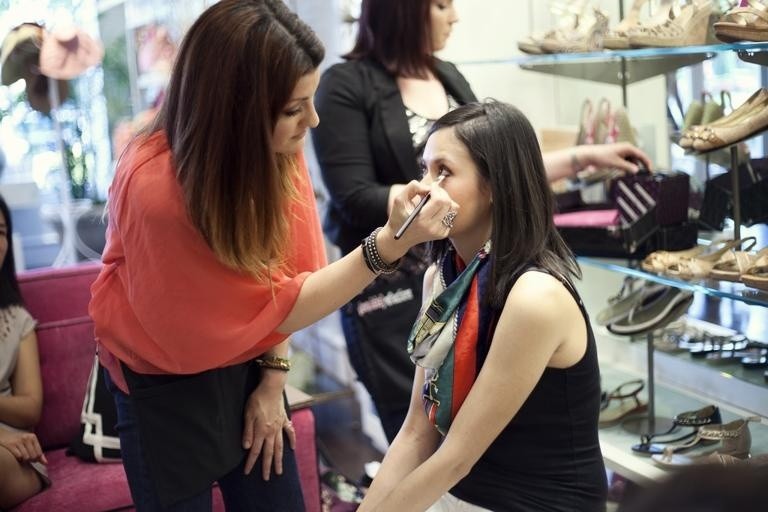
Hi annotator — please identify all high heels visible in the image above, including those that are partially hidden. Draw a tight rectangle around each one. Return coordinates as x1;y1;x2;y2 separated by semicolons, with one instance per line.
687;91;750;167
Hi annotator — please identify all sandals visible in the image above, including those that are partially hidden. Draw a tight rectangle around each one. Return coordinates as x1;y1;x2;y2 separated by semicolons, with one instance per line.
517;0;711;53
595;237;768;372
571;101;636;183
715;1;768;42
630;405;760;472
597;380;648;428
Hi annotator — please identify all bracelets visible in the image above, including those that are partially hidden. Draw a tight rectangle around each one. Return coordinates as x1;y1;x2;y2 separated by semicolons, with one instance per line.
361;236;381;276
254;355;291;374
367;224;403;275
566;148;583;174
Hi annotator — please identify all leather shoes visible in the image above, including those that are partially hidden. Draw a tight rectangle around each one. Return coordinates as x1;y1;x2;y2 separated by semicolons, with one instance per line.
678;87;768;149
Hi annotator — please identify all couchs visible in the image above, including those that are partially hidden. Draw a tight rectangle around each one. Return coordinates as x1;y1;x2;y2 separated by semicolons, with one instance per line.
1;260;324;510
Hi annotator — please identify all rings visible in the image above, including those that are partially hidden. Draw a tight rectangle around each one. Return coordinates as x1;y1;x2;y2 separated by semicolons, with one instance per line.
439;208;457;230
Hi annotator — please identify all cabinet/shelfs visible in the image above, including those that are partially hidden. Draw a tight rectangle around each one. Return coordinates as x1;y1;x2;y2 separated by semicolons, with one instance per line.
515;3;768;509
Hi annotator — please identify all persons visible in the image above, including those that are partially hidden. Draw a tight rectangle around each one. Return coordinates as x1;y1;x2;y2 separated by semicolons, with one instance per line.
1;199;55;510
85;2;461;511
356;96;606;511
307;0;665;445
